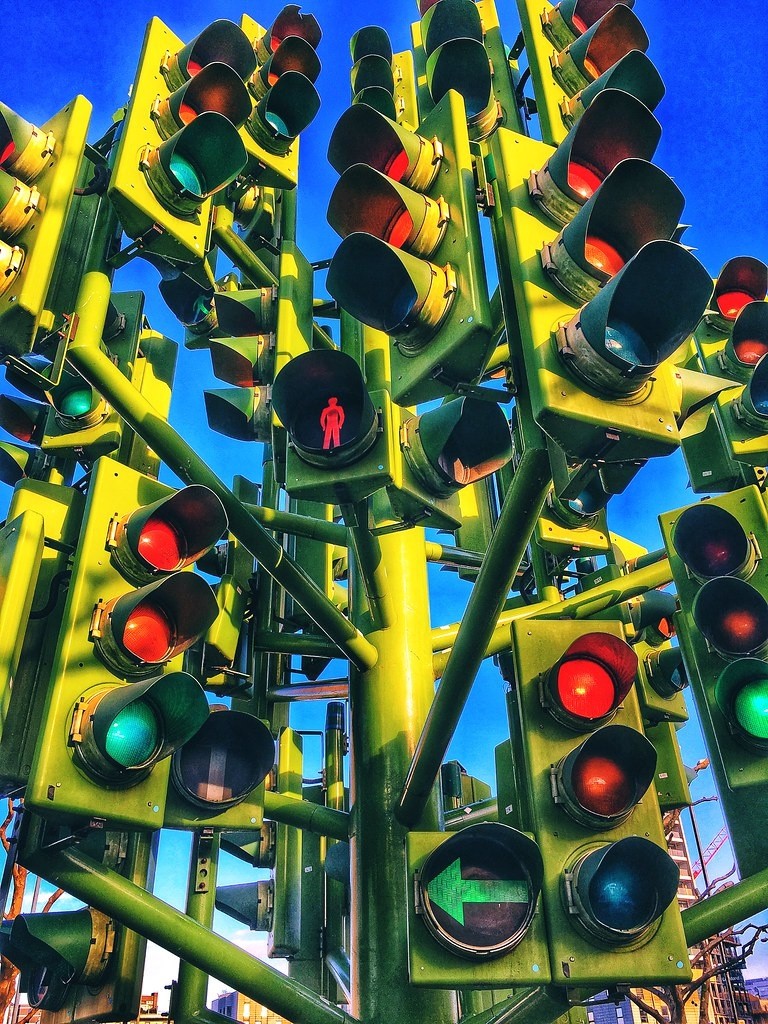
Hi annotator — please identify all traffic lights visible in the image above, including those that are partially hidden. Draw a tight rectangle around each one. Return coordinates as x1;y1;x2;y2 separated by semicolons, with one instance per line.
385;391;515;532
350;25;418;134
486;125;714;462
24;456;229;832
5;805;153;1023
214;724;304;960
411;0;524;162
107;15;257;264
159;271;240;351
271;348;395;506
534;438;613;558
41;288;145;463
0;92;95;360
326;87;493;409
203;238;314;482
657;484;768;791
604;529;692;724
517;0;665;148
406;819;555;985
509;616;697;985
690;256;768;468
0;352;78;488
240;6;324;189
162;708;275;834
